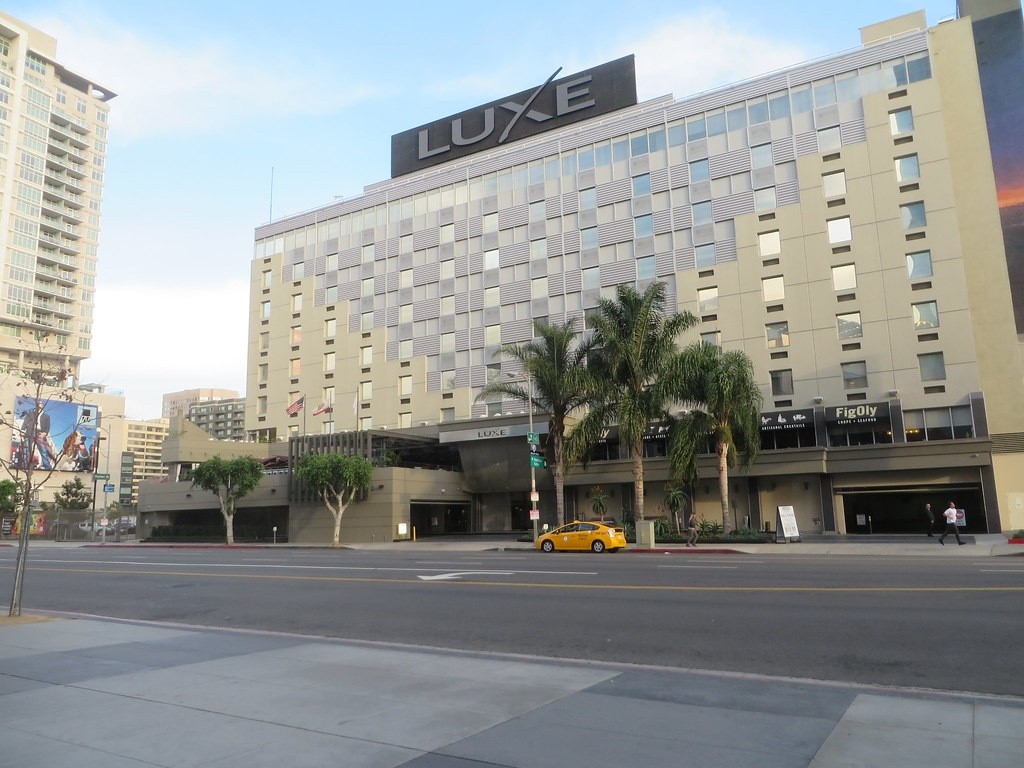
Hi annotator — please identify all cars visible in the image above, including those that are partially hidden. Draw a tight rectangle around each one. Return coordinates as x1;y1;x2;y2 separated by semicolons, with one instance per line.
536;520;626;553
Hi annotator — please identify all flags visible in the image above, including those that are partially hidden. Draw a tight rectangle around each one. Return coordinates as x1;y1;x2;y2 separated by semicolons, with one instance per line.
285;395;304;415
312;396;330;415
352;390;362;415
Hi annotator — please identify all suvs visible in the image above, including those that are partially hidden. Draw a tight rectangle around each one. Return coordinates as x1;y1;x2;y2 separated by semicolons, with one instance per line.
78;522;107;535
105;523;137;535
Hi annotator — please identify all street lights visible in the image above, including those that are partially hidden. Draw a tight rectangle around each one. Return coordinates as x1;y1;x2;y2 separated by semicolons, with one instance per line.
96;424;111;544
507;368;541;547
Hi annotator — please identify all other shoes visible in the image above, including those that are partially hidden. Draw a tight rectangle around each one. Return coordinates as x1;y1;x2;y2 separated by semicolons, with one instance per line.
692;544;698;547
938;538;944;545
686;544;691;547
927;534;934;537
959;540;967;545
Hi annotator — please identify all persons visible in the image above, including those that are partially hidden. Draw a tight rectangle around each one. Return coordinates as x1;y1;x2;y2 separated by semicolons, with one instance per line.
925;503;935;538
937;501;966;545
685;512;699;548
565;523;578;532
21;400;53;471
